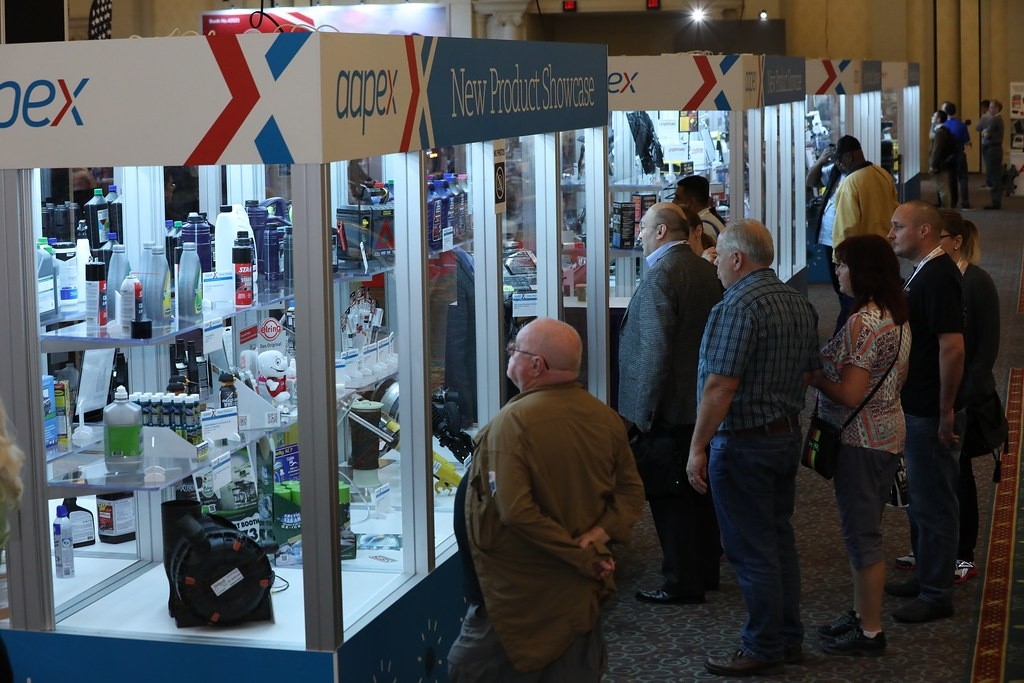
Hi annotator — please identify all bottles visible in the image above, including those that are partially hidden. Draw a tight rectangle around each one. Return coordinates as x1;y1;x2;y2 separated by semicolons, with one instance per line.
286;300;295;341
272;480;351;544
129;391;203;445
56;363;80;426
103;385;143;473
426;171;473;251
53;505;75;578
31;185;294;340
373;178;394;202
166;339;208;397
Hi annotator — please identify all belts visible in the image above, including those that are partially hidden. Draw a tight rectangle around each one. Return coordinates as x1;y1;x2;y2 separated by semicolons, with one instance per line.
715;414;797;437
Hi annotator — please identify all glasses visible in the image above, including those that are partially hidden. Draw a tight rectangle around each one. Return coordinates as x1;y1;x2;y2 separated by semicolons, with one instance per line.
506;342;550;371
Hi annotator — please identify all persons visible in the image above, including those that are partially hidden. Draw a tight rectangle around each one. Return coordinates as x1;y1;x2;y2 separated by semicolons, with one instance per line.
675;176;726;265
977;99;1005;210
926;102;975;211
615;201;726;603
422;246;477;429
801;234;912;655
685;219;824;675
446;316;645;683
806;134;899;341
887;201;1001;627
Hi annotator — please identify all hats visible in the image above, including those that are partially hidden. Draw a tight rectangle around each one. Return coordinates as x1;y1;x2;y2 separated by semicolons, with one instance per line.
831;135;861;159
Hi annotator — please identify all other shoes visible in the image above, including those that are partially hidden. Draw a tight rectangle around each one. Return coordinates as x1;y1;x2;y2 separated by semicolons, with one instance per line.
950;204;957;208
962;206;976;212
984;202;1001;210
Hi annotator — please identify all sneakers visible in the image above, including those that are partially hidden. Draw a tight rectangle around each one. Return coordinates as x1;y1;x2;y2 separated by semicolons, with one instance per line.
705;648;785;675
885;578;920;599
890;594;954;624
782;645;805;664
817;610;864;640
895;552;918;570
952;560;978;584
821;626;887;657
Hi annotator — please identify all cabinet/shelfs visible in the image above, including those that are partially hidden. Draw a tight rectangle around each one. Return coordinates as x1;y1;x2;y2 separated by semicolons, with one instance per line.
0;33;921;683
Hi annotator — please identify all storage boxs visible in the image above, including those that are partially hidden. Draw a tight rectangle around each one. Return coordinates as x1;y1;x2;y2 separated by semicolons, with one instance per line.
613;200;637;251
630;192;657;248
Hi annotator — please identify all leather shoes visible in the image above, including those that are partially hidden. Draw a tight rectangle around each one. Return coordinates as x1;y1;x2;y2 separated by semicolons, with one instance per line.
636;588;678;605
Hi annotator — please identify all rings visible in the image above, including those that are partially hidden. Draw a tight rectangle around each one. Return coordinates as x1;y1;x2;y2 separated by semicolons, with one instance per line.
691;478;695;481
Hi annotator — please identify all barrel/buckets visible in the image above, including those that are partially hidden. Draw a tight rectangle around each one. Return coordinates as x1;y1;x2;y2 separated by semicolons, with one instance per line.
95;490;136;544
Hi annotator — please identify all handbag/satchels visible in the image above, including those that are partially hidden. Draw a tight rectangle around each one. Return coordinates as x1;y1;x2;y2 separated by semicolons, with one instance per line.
805;203;823;244
885;454;910;508
802;417;842;480
960;376;1009;483
